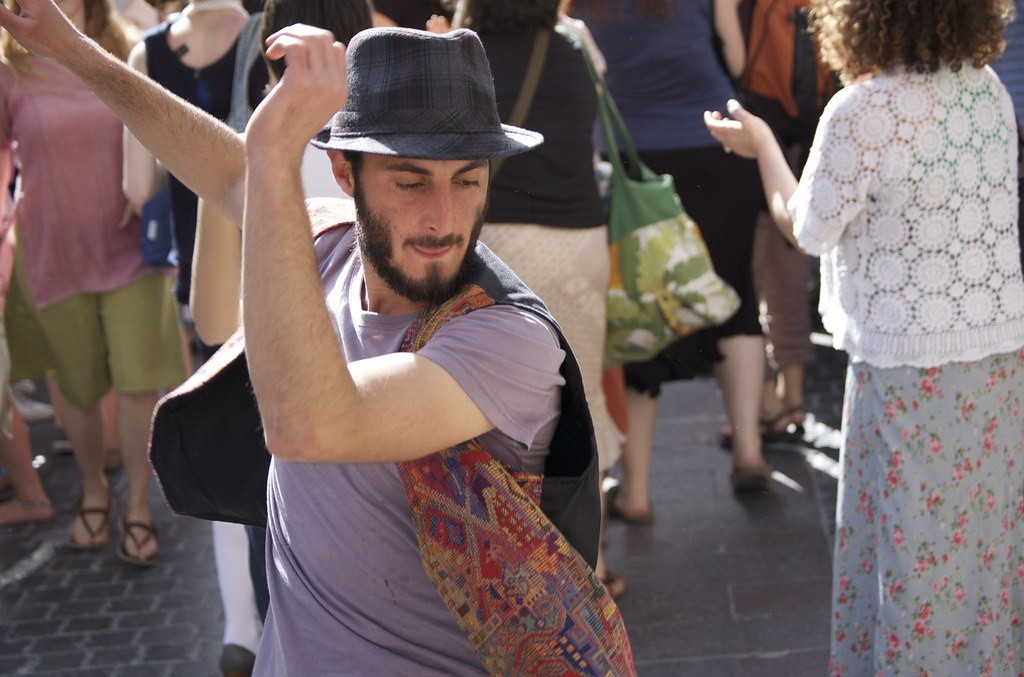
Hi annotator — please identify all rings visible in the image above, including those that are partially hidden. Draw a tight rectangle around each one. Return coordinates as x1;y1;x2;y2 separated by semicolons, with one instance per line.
723;145;732;154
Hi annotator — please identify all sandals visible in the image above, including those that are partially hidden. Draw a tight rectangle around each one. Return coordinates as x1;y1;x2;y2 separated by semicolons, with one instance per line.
718;404;805;445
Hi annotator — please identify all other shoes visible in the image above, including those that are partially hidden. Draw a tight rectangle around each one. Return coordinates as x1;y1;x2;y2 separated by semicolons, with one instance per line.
592;567;627;602
0;524;55;593
179;304;194;326
220;644;256;677
104;449;121;474
51;424;74;453
5;376;55;422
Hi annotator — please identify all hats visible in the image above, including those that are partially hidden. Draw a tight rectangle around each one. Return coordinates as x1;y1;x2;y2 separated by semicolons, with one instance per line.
310;27;545;161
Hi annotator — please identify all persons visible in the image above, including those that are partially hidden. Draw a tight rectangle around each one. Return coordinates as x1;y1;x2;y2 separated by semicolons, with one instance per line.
0;0;637;677
0;0;1024;677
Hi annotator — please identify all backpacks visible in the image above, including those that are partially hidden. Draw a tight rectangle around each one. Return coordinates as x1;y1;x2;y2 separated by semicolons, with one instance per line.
740;0;837;145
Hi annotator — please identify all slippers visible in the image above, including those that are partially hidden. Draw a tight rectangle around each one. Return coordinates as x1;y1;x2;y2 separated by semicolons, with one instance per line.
600;472;654;526
729;458;771;491
69;496;112;550
117;511;159;566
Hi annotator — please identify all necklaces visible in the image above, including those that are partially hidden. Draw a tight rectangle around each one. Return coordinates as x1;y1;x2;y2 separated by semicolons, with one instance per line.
182;1;249;18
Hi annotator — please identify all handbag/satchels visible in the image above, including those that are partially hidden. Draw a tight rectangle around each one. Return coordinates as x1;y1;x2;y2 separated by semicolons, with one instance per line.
556;26;742;368
140;188;173;267
